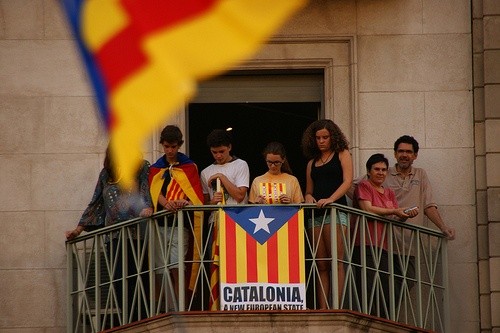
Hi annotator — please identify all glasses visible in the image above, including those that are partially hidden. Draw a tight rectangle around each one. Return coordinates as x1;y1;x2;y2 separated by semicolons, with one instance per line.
267;160;282;166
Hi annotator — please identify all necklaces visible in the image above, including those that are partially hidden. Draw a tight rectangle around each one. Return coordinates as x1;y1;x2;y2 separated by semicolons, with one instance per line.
320;150;333;164
108;170;124;184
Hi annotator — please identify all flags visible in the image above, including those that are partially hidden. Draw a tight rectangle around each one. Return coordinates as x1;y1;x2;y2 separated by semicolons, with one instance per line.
208;177;225;311
219;206;306;311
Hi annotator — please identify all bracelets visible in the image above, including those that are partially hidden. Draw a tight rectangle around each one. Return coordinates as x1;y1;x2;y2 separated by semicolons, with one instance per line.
74;229;80;236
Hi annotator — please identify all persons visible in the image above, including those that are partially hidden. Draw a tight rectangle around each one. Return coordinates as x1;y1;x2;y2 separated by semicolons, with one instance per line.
248;144;310;311
345;135;456;319
66;135;153;324
199;128;249;310
350;153;419;318
149;125;204;313
305;120;352;310
